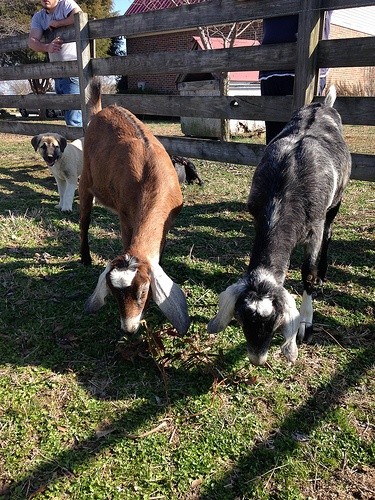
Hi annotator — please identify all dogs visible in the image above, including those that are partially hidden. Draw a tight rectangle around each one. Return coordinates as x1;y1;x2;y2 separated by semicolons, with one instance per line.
31;132;86;215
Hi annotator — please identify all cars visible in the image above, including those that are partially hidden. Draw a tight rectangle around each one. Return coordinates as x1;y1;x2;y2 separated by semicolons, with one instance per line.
18;92;63;118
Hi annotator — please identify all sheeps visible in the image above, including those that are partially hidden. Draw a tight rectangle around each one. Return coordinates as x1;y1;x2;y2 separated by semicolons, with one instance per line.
205;84;352;370
79;103;193;340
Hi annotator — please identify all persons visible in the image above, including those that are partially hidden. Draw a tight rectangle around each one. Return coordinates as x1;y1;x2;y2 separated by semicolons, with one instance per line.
29;0;83;128
258;10;332;145
